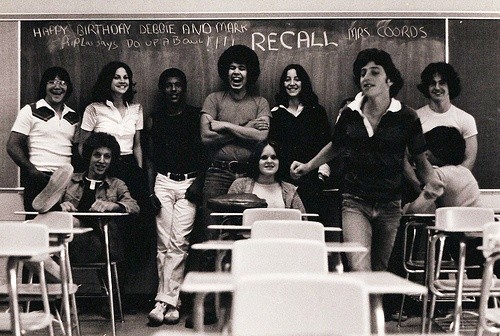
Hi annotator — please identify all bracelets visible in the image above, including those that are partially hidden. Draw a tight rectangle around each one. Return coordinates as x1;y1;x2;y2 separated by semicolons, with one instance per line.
193;177;204;186
146;193;155;198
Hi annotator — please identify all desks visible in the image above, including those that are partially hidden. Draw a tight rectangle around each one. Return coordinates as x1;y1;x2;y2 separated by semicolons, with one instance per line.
12;213;130;336
421;226;483;336
210;213;321;240
191;243;369;335
396;213;436;336
0;246;65;336
207;225;342;254
48;228;94;336
180;271;428;335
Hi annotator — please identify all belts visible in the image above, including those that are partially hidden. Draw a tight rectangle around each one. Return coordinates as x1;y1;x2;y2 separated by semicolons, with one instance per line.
160;169;200;182
211;158;260;173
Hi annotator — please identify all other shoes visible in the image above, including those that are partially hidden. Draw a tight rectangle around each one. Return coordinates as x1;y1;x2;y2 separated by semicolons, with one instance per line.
390;304;408;321
101;306;123;321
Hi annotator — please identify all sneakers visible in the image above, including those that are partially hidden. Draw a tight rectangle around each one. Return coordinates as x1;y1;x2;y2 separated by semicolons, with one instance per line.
32;163;74;213
147;297;181;327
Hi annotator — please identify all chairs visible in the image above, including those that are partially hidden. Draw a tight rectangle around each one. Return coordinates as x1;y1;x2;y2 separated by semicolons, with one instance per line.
0;192;499;336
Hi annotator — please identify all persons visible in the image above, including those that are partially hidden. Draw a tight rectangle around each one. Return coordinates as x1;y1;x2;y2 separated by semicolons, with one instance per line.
403;127;484;284
290;49;447;271
391;62;478;320
6;67;80;212
78;61;144;319
270;65;330;214
228;138;307;220
143;68;210;323
185;45;272;328
32;133;140;262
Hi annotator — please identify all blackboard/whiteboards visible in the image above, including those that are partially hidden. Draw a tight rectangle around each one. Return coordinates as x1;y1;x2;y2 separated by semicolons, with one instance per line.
16;17;500;198
